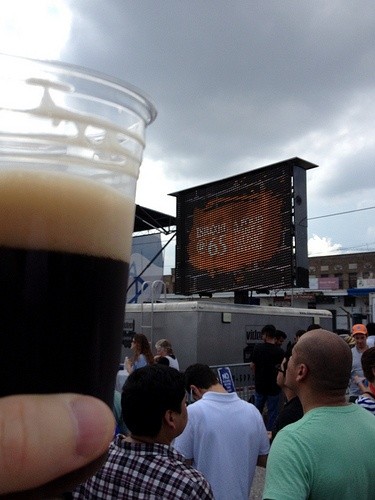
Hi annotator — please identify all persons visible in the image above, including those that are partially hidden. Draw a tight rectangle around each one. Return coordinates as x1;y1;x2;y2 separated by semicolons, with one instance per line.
113;333;179;423
170;363;270;500
71;365;214;499
1;394;116;489
250;322;375;500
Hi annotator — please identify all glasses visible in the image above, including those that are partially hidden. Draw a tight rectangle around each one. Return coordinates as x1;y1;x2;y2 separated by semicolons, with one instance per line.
189;389;195;402
131;339;136;344
274;363;284;373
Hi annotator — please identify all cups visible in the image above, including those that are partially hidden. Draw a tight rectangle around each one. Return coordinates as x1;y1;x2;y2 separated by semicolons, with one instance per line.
1;49;157;491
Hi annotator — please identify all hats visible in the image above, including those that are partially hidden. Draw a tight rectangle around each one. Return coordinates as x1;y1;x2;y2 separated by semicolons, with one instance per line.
351;324;368;337
